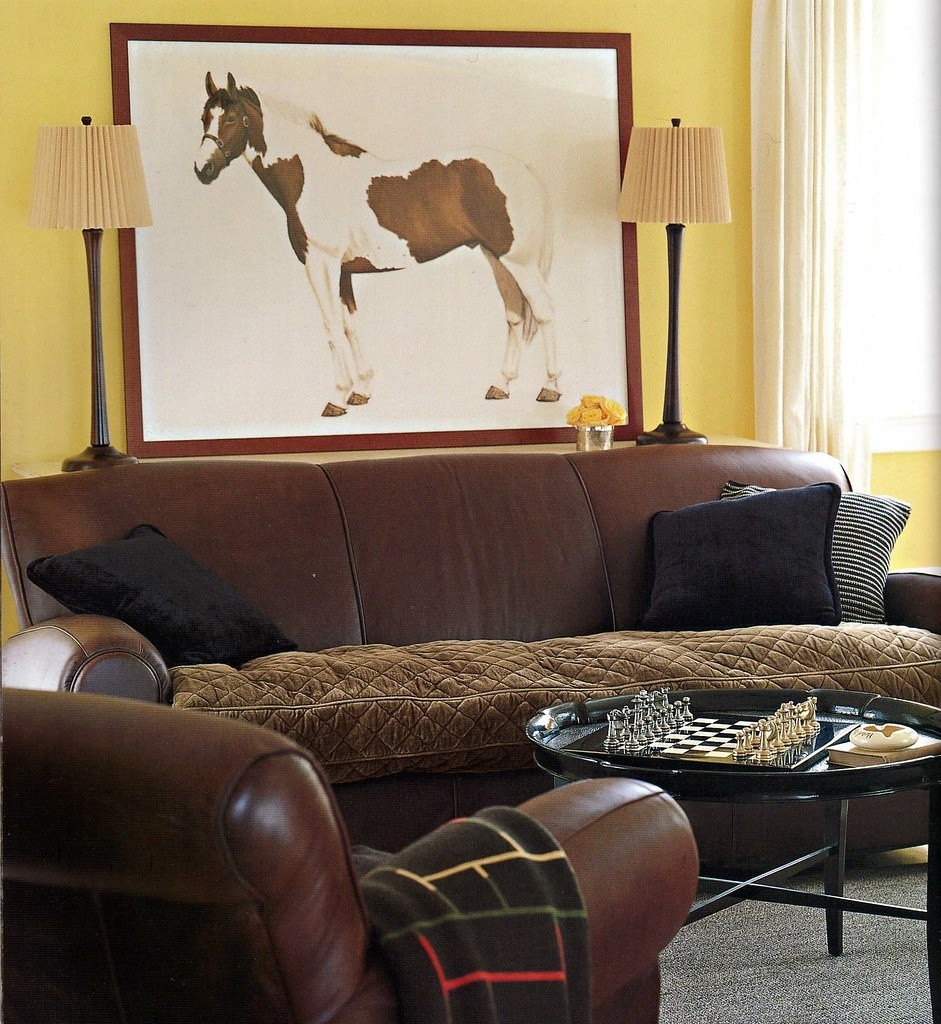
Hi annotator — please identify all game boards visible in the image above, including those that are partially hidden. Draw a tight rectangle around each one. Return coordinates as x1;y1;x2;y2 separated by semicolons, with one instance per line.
603;715;819;763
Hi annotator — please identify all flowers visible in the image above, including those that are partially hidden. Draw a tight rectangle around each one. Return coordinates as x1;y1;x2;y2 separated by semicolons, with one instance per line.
566;394;628;428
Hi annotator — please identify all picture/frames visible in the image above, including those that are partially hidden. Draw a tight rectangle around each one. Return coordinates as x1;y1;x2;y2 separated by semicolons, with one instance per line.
107;22;648;459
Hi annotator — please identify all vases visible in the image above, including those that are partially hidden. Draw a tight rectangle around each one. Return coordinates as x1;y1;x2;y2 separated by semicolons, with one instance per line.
576;425;616;452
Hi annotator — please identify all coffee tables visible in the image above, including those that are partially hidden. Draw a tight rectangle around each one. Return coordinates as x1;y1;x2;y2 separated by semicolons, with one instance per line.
524;689;941;1024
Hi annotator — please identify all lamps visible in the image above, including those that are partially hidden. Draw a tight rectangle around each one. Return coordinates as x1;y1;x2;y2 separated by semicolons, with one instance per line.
621;119;736;450
26;116;157;467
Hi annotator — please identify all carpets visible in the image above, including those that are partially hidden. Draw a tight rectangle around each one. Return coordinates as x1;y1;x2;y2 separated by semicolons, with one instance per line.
659;862;933;1024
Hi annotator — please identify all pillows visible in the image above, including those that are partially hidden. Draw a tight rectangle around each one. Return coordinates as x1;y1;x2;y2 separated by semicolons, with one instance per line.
719;479;914;626
633;481;844;628
28;521;300;669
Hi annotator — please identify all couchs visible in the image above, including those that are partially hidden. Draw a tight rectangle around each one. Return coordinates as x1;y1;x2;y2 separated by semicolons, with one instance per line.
0;440;941;879
1;687;705;1024
